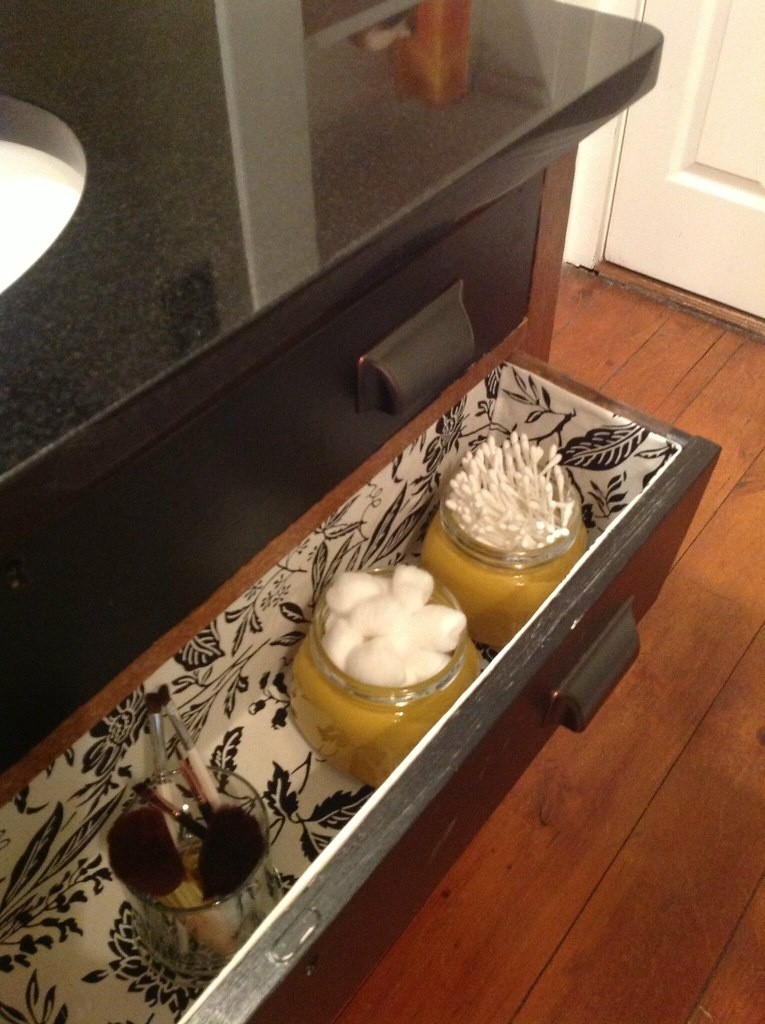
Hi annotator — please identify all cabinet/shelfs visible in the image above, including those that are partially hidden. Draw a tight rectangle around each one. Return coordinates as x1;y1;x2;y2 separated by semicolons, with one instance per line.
0;2;724;1024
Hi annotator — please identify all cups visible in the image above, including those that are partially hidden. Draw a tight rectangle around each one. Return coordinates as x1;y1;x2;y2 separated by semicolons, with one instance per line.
123;767;283;974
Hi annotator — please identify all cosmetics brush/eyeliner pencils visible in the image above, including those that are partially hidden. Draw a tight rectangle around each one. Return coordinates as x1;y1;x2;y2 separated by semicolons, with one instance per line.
106;685;266;967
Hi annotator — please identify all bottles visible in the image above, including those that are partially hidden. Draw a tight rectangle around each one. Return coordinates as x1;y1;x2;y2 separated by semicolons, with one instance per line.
424;465;586;654
289;567;480;786
